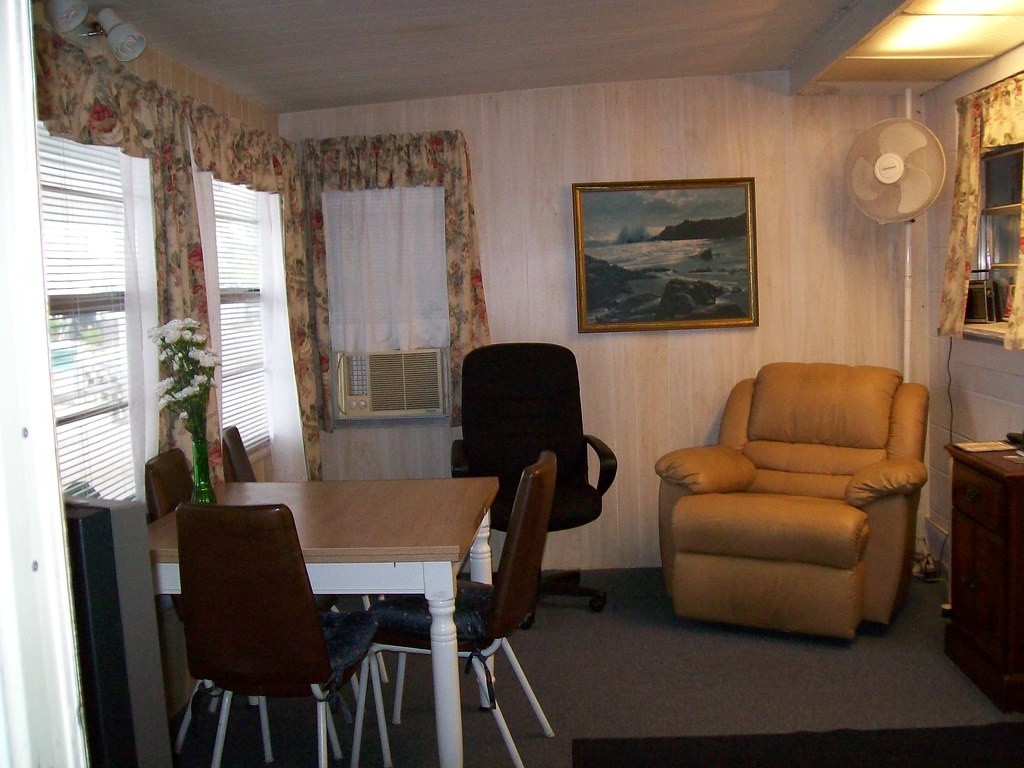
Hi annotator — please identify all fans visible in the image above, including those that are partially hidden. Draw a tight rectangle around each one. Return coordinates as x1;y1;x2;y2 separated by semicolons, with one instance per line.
844;118;946;224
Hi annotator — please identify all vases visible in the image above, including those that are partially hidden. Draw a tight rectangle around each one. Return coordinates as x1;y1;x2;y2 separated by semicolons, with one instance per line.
191;435;217;505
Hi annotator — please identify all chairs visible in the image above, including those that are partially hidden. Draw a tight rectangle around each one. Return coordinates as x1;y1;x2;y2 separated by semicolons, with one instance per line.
146;343;617;768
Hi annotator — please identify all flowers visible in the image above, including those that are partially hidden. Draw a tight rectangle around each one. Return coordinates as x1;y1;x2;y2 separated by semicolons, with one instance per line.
146;318;220;439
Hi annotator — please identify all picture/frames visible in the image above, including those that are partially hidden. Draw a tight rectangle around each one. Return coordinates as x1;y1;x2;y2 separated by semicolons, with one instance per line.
571;176;759;335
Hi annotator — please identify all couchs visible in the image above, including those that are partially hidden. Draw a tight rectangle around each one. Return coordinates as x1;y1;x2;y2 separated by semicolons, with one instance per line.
654;363;929;639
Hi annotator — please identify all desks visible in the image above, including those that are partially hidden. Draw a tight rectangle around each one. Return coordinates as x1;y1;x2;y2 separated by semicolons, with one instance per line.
146;477;498;768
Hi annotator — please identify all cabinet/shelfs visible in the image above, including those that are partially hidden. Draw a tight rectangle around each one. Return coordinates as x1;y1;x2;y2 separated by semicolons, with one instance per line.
942;441;1024;712
